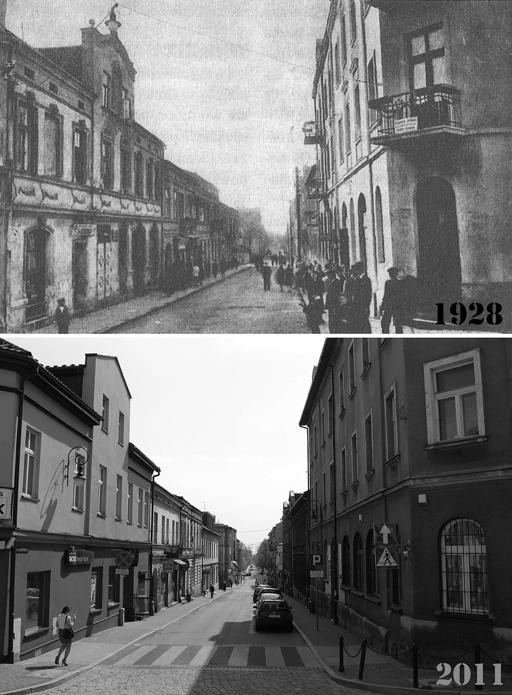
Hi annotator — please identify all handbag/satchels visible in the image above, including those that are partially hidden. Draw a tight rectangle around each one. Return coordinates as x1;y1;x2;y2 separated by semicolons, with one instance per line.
63;616;74;640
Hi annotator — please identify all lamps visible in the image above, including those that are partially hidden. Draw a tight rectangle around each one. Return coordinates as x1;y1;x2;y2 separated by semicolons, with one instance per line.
62;445;90;487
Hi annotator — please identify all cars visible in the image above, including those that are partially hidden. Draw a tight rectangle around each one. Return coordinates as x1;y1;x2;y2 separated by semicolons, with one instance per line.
254;585;295;634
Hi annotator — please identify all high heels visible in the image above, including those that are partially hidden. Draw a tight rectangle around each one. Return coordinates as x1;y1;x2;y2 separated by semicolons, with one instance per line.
62;659;68;666
55;656;59;664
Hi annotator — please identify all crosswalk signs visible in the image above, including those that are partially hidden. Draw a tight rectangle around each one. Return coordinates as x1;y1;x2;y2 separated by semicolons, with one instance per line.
374;548;399;569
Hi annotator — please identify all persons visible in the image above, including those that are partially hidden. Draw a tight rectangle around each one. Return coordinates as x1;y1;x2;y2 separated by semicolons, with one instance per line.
54;605;75;665
51;249;421;334
201;579;233;598
